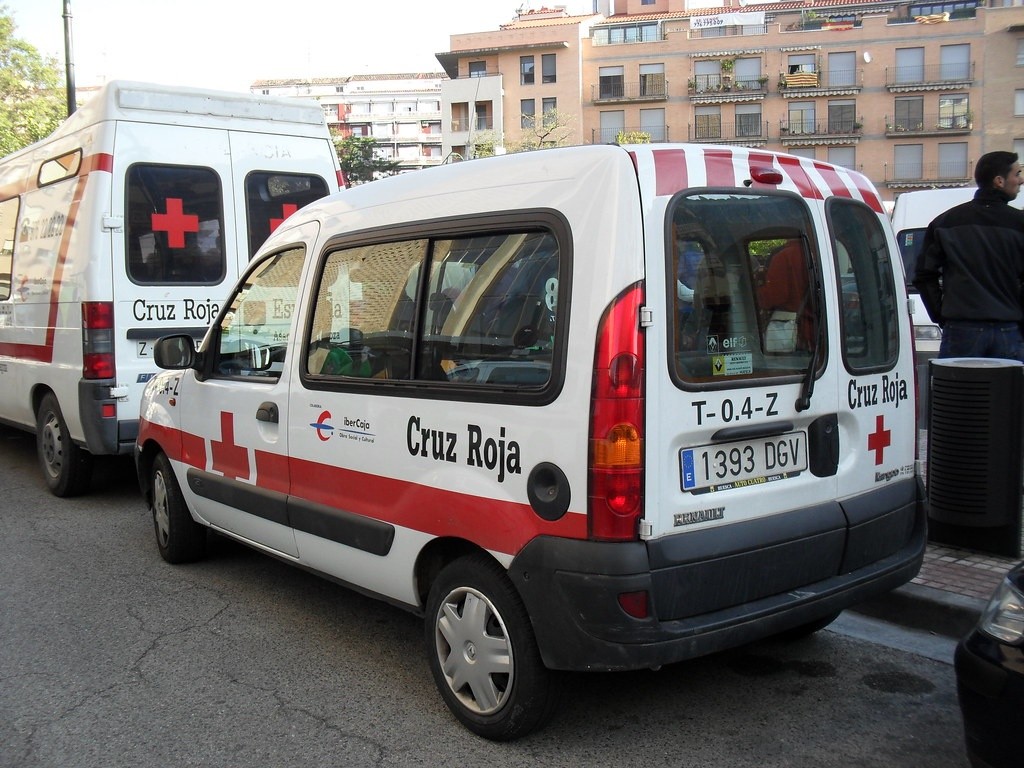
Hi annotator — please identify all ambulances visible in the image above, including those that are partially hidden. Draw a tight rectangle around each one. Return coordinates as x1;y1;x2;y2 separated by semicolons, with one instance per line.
130;145;930;743
0;77;347;497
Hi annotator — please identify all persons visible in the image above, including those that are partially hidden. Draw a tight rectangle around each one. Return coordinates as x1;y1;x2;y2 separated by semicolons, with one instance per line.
915;150;1024;359
755;236;818;353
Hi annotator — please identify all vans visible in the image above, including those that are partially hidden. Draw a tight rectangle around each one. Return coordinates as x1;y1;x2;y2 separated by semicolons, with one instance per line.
890;186;1024;431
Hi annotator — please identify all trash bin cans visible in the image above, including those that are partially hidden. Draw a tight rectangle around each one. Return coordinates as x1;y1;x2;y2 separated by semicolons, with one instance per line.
926;356;1024;557
953;559;1023;768
